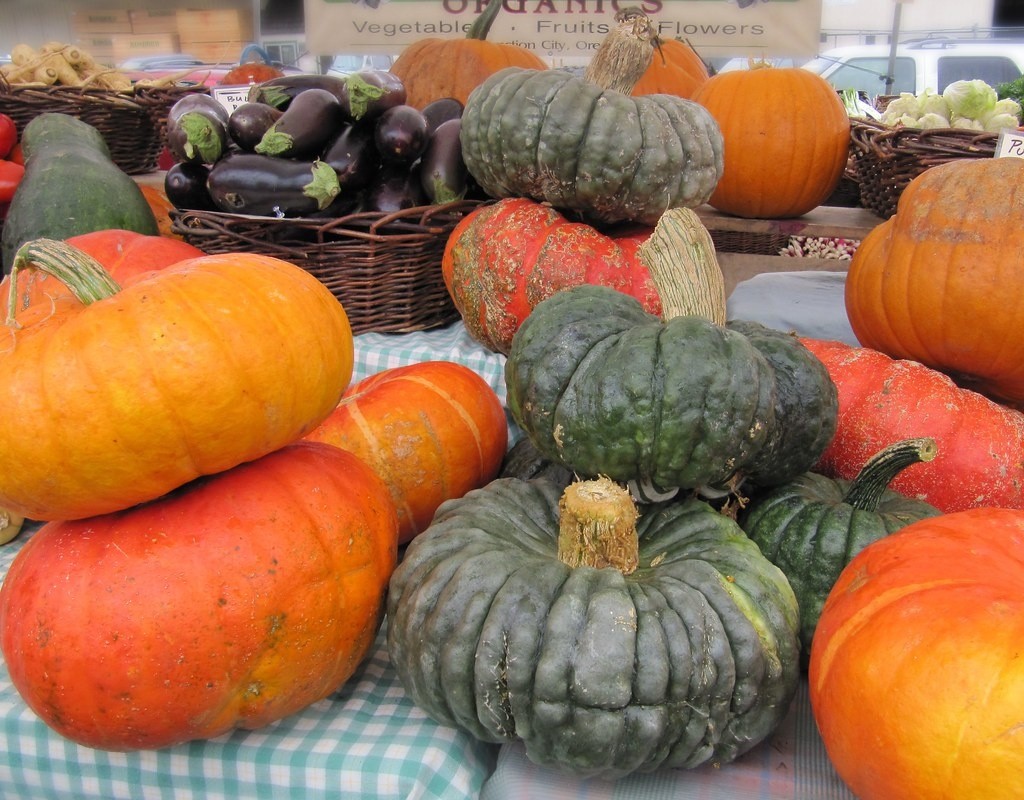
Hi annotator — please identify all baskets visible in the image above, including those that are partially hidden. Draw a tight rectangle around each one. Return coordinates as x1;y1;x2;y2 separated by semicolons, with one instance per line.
169;200;499;335
0;76;211;173
845;116;1000;221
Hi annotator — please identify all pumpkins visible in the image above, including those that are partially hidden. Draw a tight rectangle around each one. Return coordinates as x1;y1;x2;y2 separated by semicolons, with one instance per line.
1;1;1024;800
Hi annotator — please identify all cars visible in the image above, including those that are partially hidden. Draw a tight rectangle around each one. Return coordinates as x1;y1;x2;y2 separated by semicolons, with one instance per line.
327;54;400;77
120;54;196;69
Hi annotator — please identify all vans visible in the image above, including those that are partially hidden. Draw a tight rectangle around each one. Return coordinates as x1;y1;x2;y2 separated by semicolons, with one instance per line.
801;42;1024;97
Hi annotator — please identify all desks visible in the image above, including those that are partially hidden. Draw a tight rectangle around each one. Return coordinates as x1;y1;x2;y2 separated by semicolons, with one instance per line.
0;193;881;800
